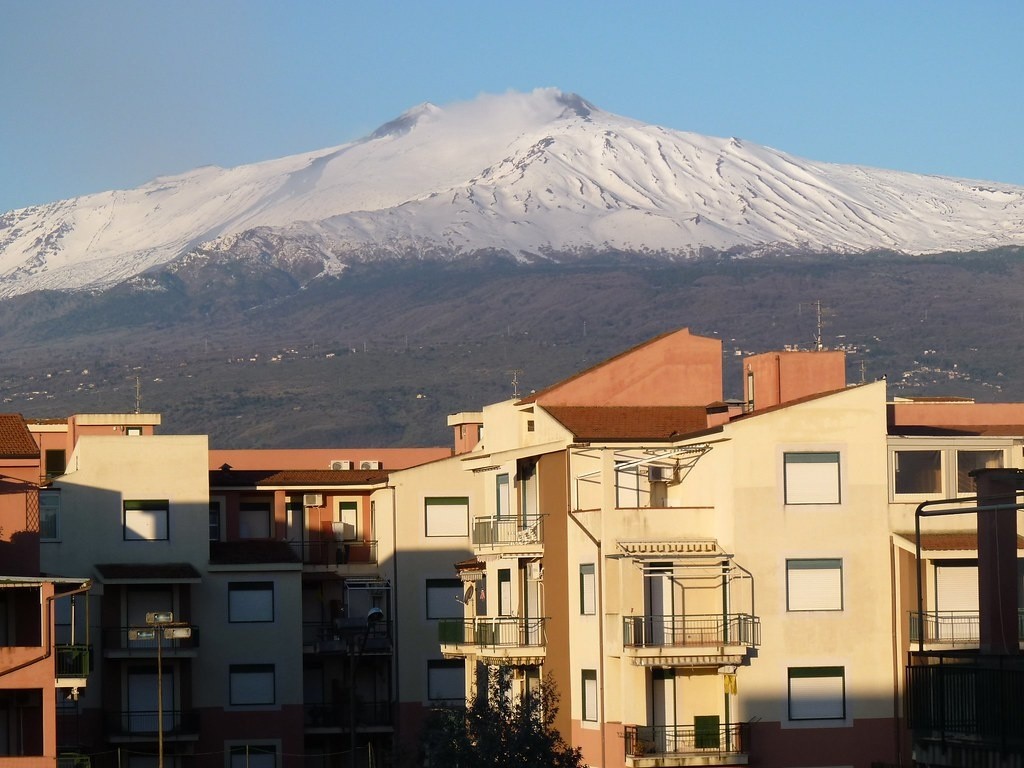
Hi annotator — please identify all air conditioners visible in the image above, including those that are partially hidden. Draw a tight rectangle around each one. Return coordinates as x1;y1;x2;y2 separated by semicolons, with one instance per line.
329;460;351;471
526;563;541;581
302;493;324;507
359;461;379;470
649;466;674;484
